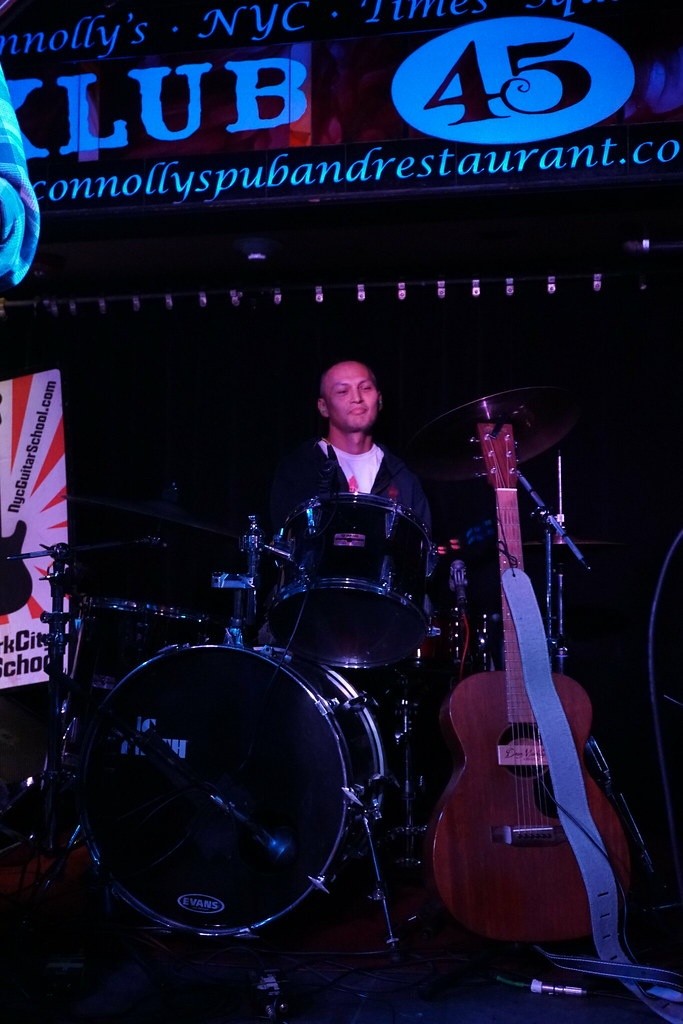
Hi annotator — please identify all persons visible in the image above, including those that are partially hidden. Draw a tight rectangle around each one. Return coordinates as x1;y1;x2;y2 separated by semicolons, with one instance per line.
270;361;432;555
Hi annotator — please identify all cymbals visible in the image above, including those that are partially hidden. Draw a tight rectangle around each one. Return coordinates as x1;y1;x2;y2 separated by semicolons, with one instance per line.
402;383;583;484
517;529;622;550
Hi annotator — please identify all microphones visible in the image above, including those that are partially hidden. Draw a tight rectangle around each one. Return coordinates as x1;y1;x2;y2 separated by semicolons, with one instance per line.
450;559;469;615
263;833;304;873
326;445;341;492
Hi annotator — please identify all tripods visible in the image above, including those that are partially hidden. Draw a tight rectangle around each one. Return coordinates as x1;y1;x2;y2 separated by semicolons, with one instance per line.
334;665;430;897
0;523;169;860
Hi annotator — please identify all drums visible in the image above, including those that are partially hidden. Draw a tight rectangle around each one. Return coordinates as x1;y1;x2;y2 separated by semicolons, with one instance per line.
265;490;438;670
52;593;210;691
76;640;387;944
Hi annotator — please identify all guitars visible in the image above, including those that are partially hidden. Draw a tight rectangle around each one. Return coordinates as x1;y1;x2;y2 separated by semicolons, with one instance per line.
416;419;636;950
